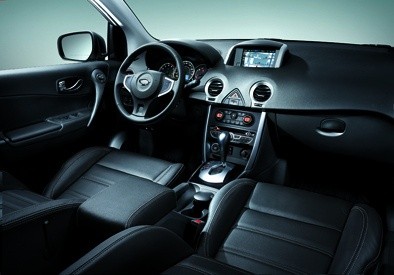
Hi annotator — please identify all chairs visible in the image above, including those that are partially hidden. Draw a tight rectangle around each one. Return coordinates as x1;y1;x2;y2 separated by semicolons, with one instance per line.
2;141;182;241
62;174;387;274
